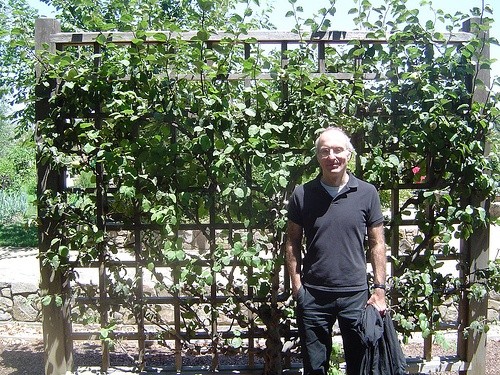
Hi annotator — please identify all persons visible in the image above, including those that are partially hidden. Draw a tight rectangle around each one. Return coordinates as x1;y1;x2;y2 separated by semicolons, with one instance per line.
284;128;388;375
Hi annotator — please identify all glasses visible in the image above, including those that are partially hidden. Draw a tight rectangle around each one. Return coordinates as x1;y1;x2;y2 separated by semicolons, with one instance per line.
318;147;348;157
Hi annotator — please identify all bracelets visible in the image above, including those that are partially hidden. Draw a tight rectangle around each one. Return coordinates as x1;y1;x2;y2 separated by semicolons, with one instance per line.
373;283;387;291
